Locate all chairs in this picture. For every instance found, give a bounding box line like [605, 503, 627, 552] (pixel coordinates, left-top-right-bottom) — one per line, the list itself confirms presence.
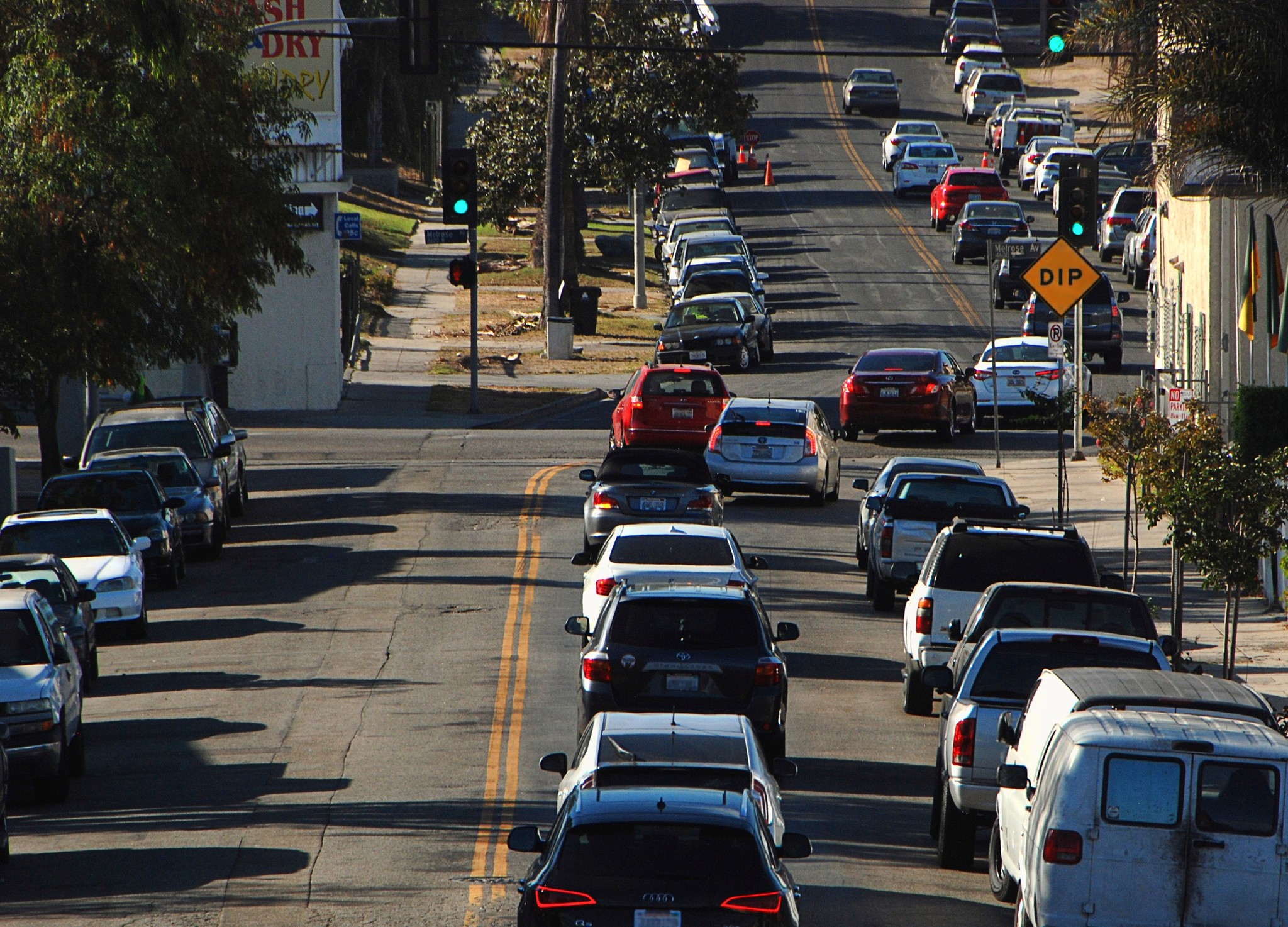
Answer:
[26, 579, 54, 602]
[691, 380, 709, 395]
[156, 461, 182, 486]
[996, 350, 1011, 361]
[936, 153, 960, 157]
[682, 314, 696, 324]
[879, 75, 889, 83]
[912, 149, 921, 156]
[621, 465, 644, 475]
[997, 611, 1032, 629]
[1217, 771, 1273, 827]
[720, 307, 734, 320]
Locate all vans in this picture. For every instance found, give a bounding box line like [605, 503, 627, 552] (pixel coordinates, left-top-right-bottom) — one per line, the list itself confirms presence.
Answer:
[985, 667, 1288, 926]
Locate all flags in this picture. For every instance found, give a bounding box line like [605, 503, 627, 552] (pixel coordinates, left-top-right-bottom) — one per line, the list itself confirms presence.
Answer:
[1238, 204, 1262, 342]
[1265, 214, 1288, 354]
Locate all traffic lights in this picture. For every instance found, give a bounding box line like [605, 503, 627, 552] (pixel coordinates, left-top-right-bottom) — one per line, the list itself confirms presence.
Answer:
[1045, 0, 1081, 64]
[443, 148, 479, 223]
[448, 260, 471, 286]
[1059, 177, 1098, 246]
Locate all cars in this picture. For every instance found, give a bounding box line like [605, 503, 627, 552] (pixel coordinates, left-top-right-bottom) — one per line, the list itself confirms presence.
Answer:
[1018, 137, 1156, 290]
[880, 119, 1035, 264]
[505, 362, 848, 927]
[646, 128, 775, 371]
[840, 337, 1094, 441]
[928, 0, 1028, 146]
[842, 68, 903, 116]
[851, 454, 989, 569]
[0, 397, 253, 797]
[509, 361, 845, 927]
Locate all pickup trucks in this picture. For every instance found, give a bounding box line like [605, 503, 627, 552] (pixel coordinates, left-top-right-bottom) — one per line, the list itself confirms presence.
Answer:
[863, 471, 1031, 613]
[916, 582, 1185, 867]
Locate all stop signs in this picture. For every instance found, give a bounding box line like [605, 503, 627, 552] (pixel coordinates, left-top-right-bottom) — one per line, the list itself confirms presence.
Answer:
[743, 130, 761, 146]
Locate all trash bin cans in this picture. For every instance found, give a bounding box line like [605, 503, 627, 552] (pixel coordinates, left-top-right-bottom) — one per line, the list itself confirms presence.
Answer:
[568, 285, 602, 337]
[546, 317, 574, 360]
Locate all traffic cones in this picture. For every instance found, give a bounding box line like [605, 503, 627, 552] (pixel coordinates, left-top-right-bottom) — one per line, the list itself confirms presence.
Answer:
[981, 150, 989, 167]
[1019, 126, 1026, 143]
[736, 143, 758, 169]
[764, 154, 776, 187]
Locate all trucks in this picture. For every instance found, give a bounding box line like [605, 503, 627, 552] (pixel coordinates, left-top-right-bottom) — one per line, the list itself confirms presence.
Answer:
[999, 95, 1081, 177]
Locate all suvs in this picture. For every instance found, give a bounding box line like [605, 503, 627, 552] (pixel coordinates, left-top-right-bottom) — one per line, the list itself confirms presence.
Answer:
[1022, 270, 1129, 368]
[901, 515, 1126, 717]
